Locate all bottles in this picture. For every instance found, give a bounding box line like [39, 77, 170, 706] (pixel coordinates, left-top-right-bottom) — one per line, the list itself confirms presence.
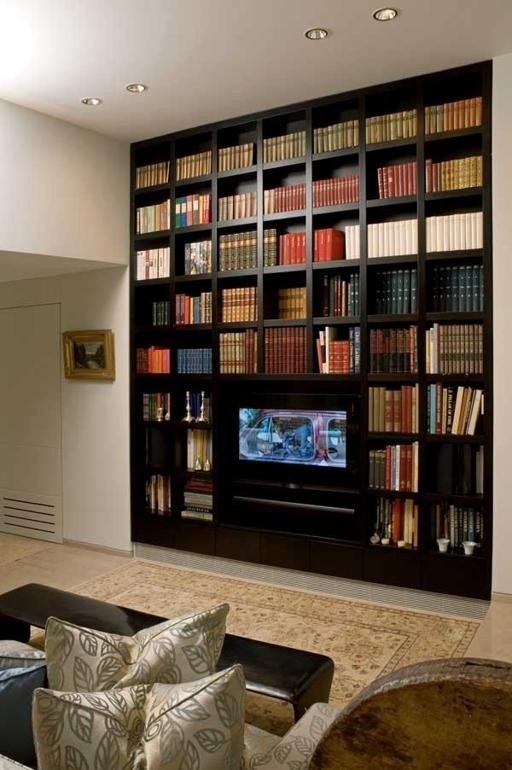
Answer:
[370, 533, 380, 544]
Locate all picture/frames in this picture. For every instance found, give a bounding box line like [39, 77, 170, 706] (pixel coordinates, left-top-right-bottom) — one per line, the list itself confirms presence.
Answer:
[59, 324, 120, 384]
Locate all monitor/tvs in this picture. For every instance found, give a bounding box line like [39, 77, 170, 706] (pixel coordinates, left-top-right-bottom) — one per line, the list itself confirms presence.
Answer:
[232, 394, 361, 494]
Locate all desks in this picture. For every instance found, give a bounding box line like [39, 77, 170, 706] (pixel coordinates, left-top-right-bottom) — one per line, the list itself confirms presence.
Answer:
[0, 578, 333, 725]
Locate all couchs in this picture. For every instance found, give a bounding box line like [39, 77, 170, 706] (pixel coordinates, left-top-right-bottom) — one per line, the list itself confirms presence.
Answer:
[0, 632, 344, 769]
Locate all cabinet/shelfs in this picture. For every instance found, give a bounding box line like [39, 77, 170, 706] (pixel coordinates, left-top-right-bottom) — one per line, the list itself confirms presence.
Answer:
[122, 54, 496, 606]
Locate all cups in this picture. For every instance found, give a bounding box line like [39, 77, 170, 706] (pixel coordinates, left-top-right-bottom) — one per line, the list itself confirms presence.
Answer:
[435, 538, 451, 554]
[398, 540, 406, 548]
[381, 538, 390, 545]
[461, 540, 476, 557]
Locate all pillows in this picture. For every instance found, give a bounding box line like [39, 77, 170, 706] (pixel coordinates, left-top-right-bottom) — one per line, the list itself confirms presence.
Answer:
[40, 603, 230, 694]
[23, 658, 252, 769]
[0, 657, 46, 769]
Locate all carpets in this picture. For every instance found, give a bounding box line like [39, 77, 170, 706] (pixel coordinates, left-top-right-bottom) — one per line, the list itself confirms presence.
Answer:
[60, 558, 482, 706]
